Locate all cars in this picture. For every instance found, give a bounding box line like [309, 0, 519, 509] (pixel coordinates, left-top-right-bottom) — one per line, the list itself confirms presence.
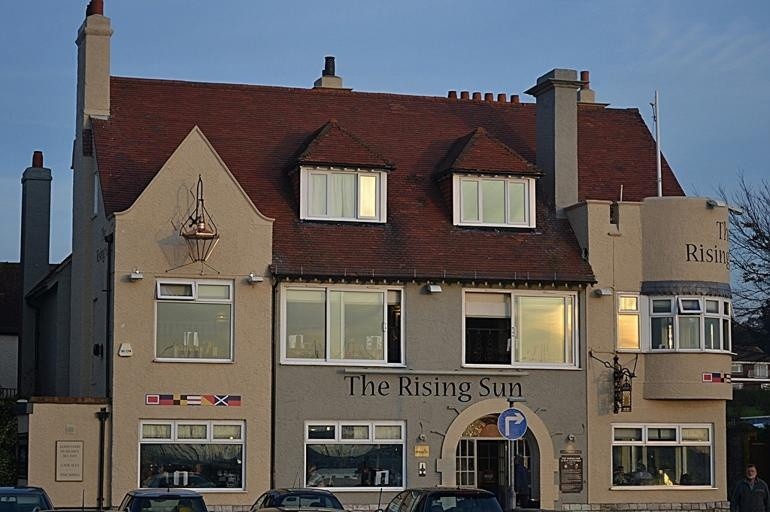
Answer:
[249, 488, 349, 512]
[226, 475, 236, 483]
[373, 485, 504, 511]
[117, 486, 209, 511]
[306, 466, 365, 487]
[143, 472, 214, 490]
[0, 486, 55, 511]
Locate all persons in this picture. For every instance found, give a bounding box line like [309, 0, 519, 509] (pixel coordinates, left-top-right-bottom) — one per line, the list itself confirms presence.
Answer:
[730, 463, 770, 512]
[306, 462, 327, 488]
[613, 453, 711, 486]
[513, 452, 531, 509]
